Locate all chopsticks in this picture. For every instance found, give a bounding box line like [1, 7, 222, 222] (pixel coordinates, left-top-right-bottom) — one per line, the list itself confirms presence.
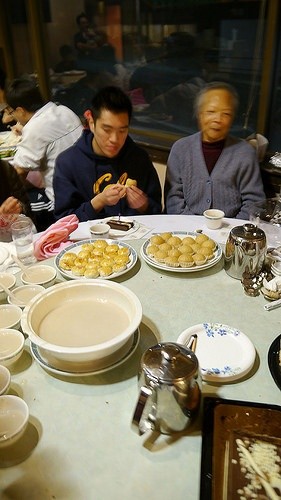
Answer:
[5, 121, 20, 141]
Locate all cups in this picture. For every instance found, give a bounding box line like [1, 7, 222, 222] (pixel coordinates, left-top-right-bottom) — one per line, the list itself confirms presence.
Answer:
[0, 396, 29, 446]
[0, 304, 22, 329]
[241, 270, 265, 296]
[21, 265, 57, 289]
[89, 224, 110, 240]
[1, 272, 45, 307]
[0, 328, 25, 366]
[203, 208, 225, 228]
[0, 365, 12, 395]
[11, 220, 38, 264]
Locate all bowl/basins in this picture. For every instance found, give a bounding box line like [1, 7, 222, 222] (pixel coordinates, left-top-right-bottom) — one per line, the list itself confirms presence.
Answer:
[270, 259, 281, 277]
[20, 279, 143, 362]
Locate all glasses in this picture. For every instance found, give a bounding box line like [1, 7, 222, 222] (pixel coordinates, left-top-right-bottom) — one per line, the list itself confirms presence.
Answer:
[8, 107, 16, 117]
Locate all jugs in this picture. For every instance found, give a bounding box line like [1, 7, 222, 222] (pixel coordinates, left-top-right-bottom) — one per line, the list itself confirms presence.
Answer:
[224, 222, 267, 279]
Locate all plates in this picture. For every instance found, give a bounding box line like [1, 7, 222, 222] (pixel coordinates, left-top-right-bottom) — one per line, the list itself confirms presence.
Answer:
[54, 238, 138, 280]
[31, 329, 141, 377]
[141, 231, 223, 271]
[99, 221, 140, 236]
[0, 131, 21, 160]
[267, 335, 281, 390]
[175, 323, 258, 383]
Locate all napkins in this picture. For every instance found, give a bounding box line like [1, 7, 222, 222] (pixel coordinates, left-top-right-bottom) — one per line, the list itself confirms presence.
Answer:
[32, 214, 80, 260]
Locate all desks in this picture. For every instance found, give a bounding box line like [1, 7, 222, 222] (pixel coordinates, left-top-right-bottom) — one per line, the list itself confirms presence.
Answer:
[0, 215, 281, 500]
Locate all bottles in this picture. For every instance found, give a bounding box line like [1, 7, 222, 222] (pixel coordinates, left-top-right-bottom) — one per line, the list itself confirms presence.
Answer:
[131, 334, 200, 437]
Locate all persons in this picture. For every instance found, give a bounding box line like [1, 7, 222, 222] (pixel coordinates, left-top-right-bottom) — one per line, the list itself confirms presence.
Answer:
[53, 86, 162, 223]
[0, 78, 84, 224]
[163, 82, 265, 221]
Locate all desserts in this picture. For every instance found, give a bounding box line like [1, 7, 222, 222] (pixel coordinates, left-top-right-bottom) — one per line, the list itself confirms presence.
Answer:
[146, 233, 217, 268]
[125, 179, 137, 188]
[60, 239, 131, 278]
